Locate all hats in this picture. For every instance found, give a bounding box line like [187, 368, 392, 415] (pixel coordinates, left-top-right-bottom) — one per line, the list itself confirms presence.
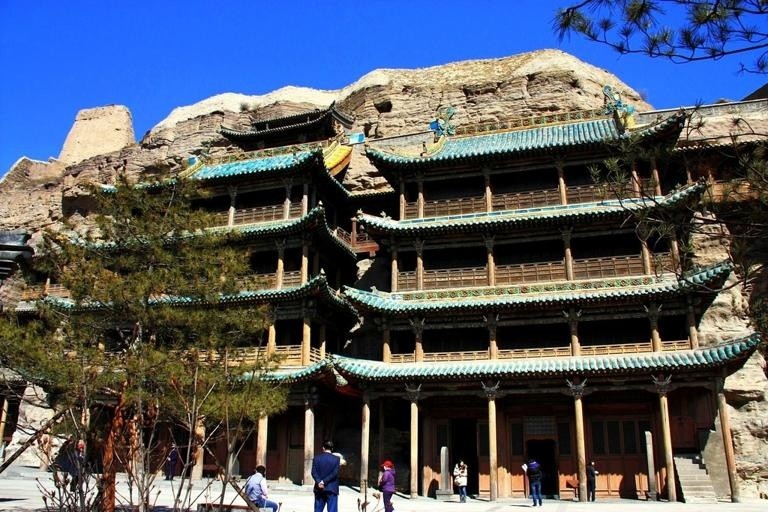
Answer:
[380, 460, 394, 468]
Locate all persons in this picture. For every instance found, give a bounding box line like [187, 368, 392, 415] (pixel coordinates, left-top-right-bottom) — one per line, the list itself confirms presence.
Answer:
[311, 440, 340, 512]
[164, 444, 176, 480]
[378, 460, 395, 512]
[55, 436, 85, 493]
[526, 458, 543, 506]
[245, 465, 278, 512]
[453, 459, 468, 503]
[586, 460, 599, 501]
